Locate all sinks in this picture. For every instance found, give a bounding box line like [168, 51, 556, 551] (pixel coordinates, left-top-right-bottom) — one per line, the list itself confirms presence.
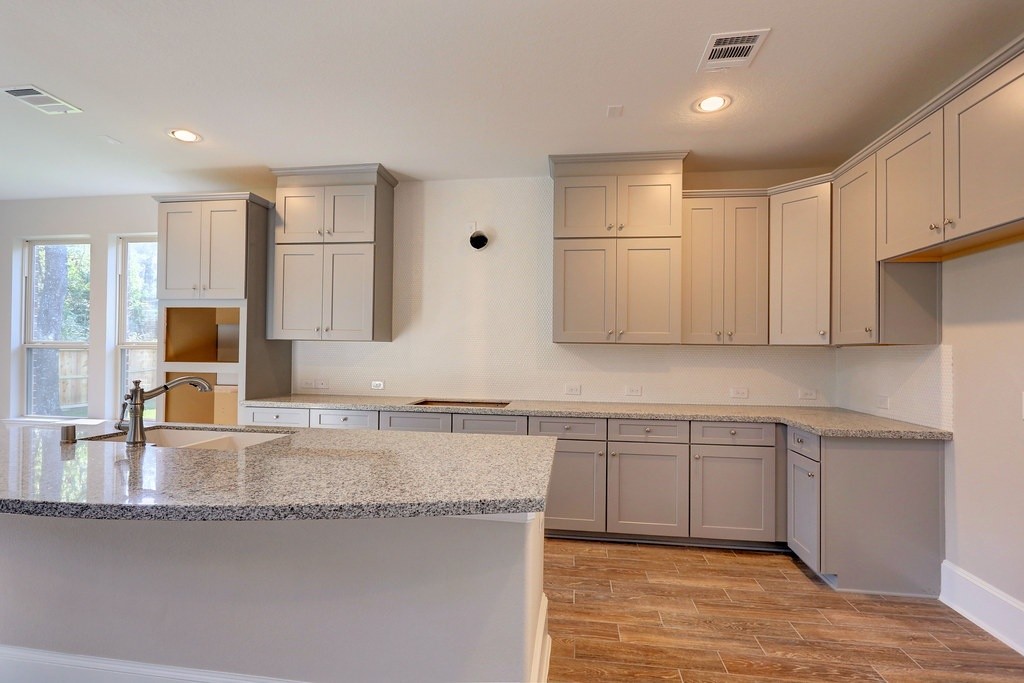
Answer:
[181, 430, 299, 450]
[78, 425, 226, 448]
[406, 397, 510, 409]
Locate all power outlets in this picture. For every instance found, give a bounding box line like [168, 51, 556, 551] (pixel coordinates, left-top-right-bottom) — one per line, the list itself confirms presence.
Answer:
[730, 386, 748, 399]
[877, 396, 890, 410]
[371, 380, 385, 389]
[564, 383, 581, 395]
[625, 384, 643, 396]
[798, 387, 818, 399]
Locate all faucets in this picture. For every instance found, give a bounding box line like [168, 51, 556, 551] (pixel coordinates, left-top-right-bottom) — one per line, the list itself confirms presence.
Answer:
[113, 374, 211, 449]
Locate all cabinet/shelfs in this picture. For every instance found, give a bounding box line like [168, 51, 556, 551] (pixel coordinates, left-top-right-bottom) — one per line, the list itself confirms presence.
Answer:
[242, 408, 380, 433]
[547, 147, 688, 238]
[787, 424, 819, 584]
[765, 185, 832, 350]
[380, 412, 528, 435]
[153, 190, 272, 424]
[267, 162, 398, 344]
[528, 417, 691, 540]
[549, 242, 682, 345]
[876, 54, 1024, 264]
[689, 422, 776, 543]
[832, 151, 941, 346]
[681, 188, 771, 345]
